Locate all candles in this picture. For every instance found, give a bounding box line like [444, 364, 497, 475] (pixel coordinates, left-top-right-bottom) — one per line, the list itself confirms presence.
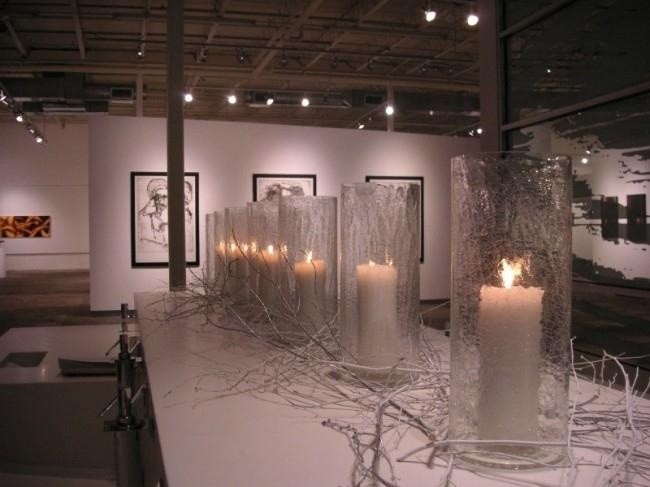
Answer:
[212, 242, 400, 381]
[470, 257, 544, 457]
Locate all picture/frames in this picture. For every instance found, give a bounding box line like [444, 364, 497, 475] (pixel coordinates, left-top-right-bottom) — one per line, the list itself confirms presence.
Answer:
[252, 172, 317, 203]
[129, 171, 201, 267]
[364, 174, 424, 264]
